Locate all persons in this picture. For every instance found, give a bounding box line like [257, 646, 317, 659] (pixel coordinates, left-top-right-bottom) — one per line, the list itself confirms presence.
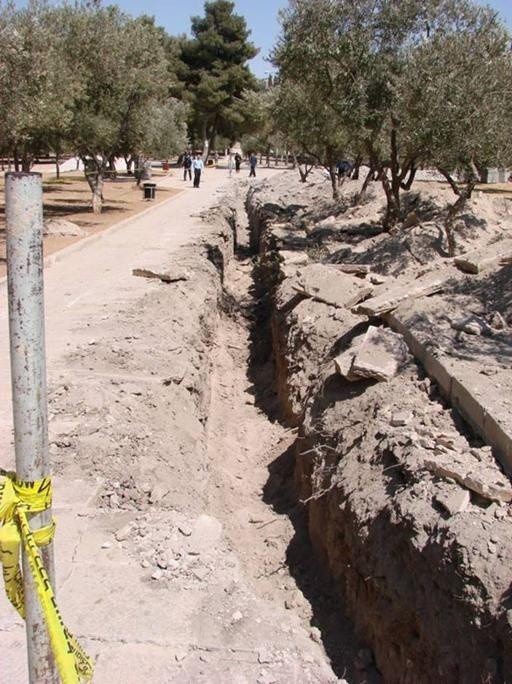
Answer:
[234, 152, 242, 172]
[247, 151, 256, 177]
[192, 153, 205, 188]
[181, 153, 193, 181]
[214, 150, 220, 165]
[227, 155, 234, 179]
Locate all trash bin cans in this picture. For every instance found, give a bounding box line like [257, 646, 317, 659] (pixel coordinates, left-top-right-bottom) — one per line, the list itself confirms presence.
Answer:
[143, 183, 155, 198]
[161, 159, 168, 169]
